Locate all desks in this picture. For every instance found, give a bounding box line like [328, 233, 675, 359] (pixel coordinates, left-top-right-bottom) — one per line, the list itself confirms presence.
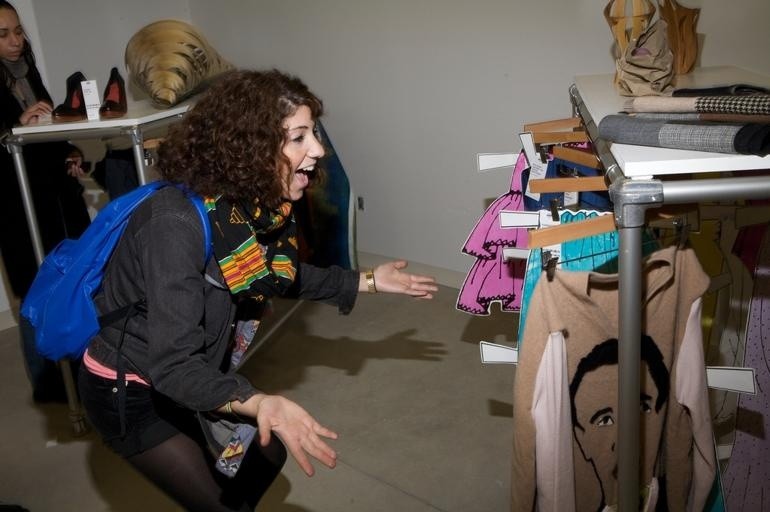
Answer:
[7, 95, 191, 448]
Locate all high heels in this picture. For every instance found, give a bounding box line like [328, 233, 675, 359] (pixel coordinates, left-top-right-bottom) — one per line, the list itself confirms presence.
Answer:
[99, 67, 127, 118]
[51, 71, 88, 125]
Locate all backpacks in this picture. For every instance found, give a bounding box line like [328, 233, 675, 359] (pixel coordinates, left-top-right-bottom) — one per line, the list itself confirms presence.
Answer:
[19, 180, 213, 362]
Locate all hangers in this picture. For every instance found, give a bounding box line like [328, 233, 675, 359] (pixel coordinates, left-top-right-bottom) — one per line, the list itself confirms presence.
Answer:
[522, 84, 701, 281]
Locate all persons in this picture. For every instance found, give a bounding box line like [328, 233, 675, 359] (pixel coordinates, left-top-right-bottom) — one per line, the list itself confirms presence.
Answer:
[0, 0, 93, 404]
[75, 67, 440, 511]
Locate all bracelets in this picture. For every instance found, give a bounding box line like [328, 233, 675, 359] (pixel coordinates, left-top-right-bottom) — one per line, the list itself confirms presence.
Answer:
[365, 269, 377, 295]
[225, 400, 233, 415]
